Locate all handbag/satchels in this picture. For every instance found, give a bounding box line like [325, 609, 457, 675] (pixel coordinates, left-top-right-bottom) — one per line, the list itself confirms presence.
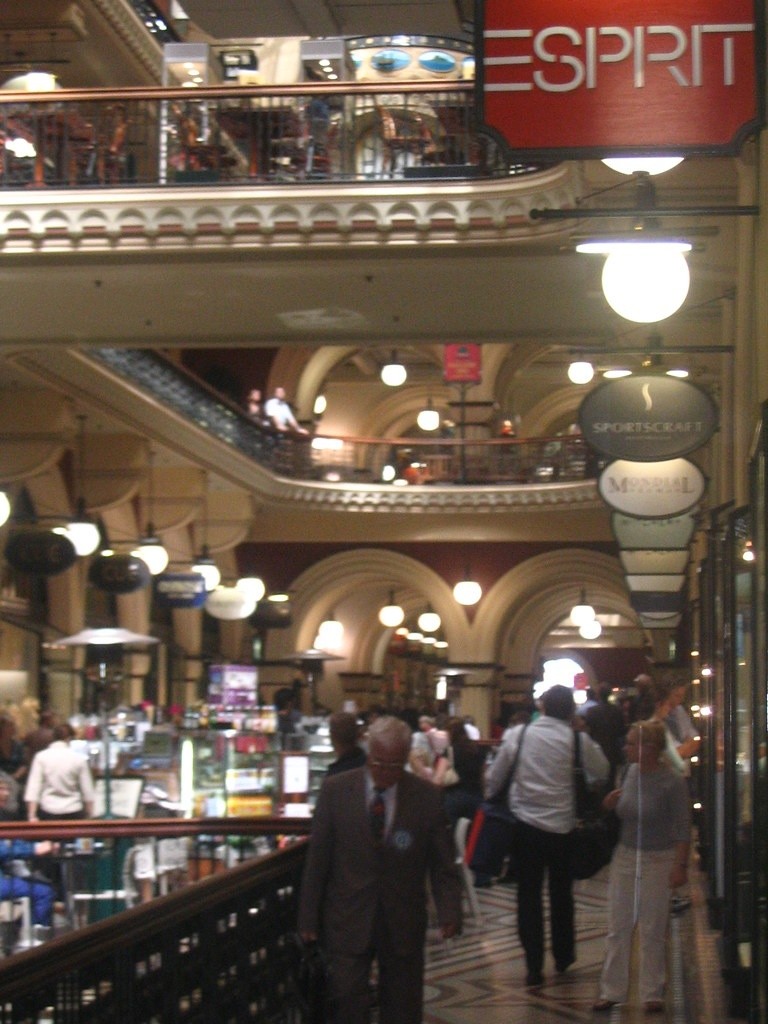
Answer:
[566, 816, 613, 879]
[439, 747, 460, 787]
[464, 803, 516, 870]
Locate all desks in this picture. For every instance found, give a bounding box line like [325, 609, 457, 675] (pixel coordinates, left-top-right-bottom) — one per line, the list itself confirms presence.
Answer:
[0, 108, 95, 187]
[207, 104, 300, 176]
[431, 99, 475, 164]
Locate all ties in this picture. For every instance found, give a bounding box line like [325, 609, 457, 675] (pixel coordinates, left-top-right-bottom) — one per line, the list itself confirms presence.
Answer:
[368, 786, 387, 842]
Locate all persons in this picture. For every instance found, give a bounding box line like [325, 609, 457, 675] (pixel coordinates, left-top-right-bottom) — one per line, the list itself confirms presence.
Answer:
[483, 686, 608, 986]
[0, 671, 704, 934]
[240, 387, 278, 428]
[261, 387, 308, 470]
[595, 719, 693, 1010]
[295, 716, 466, 1024]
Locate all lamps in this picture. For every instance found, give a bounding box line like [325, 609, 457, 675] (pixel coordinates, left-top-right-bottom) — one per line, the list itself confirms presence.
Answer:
[569, 588, 602, 641]
[576, 170, 692, 322]
[568, 353, 594, 384]
[380, 351, 407, 387]
[45, 415, 266, 620]
[417, 398, 441, 431]
[378, 558, 485, 648]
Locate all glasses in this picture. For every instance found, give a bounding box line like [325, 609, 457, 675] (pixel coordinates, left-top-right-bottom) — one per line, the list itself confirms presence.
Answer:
[369, 762, 403, 773]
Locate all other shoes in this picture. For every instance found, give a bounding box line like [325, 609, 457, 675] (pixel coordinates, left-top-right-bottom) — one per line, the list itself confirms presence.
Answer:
[669, 895, 690, 912]
[647, 1001, 664, 1011]
[594, 998, 617, 1010]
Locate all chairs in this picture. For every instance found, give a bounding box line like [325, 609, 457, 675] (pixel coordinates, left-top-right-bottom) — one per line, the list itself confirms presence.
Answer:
[68, 92, 427, 184]
